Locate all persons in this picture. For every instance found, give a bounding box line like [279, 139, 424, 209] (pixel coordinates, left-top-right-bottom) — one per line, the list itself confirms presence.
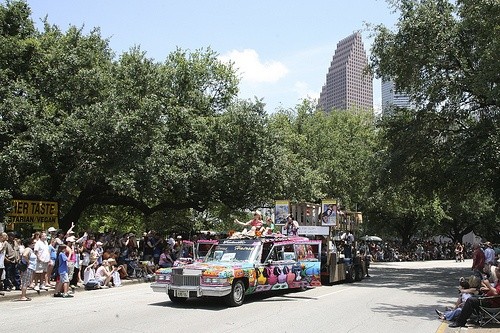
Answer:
[232, 210, 264, 233]
[0, 219, 500, 329]
[264, 215, 299, 237]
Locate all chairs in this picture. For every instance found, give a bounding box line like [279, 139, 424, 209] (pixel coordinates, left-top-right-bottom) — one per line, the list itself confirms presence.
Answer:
[478, 295, 500, 326]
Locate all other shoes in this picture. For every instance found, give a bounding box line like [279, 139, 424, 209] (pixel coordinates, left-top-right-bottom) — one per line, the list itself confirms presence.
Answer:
[53, 291, 63, 297]
[449, 320, 466, 327]
[39, 284, 48, 290]
[44, 284, 52, 287]
[15, 287, 21, 291]
[19, 296, 32, 301]
[63, 293, 74, 298]
[434, 308, 446, 320]
[49, 282, 57, 286]
[366, 275, 371, 277]
[34, 286, 40, 292]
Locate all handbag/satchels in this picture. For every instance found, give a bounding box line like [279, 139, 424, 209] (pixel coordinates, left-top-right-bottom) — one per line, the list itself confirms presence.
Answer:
[16, 260, 28, 272]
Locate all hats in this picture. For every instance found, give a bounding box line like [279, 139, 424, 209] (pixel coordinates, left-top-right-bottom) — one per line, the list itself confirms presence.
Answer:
[483, 241, 492, 248]
[96, 241, 104, 246]
[70, 235, 78, 240]
[47, 226, 57, 232]
[67, 230, 75, 234]
[66, 237, 76, 243]
[108, 257, 116, 261]
[251, 210, 262, 220]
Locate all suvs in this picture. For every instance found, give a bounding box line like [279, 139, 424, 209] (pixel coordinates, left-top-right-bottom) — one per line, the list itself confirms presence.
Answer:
[150, 236, 322, 305]
